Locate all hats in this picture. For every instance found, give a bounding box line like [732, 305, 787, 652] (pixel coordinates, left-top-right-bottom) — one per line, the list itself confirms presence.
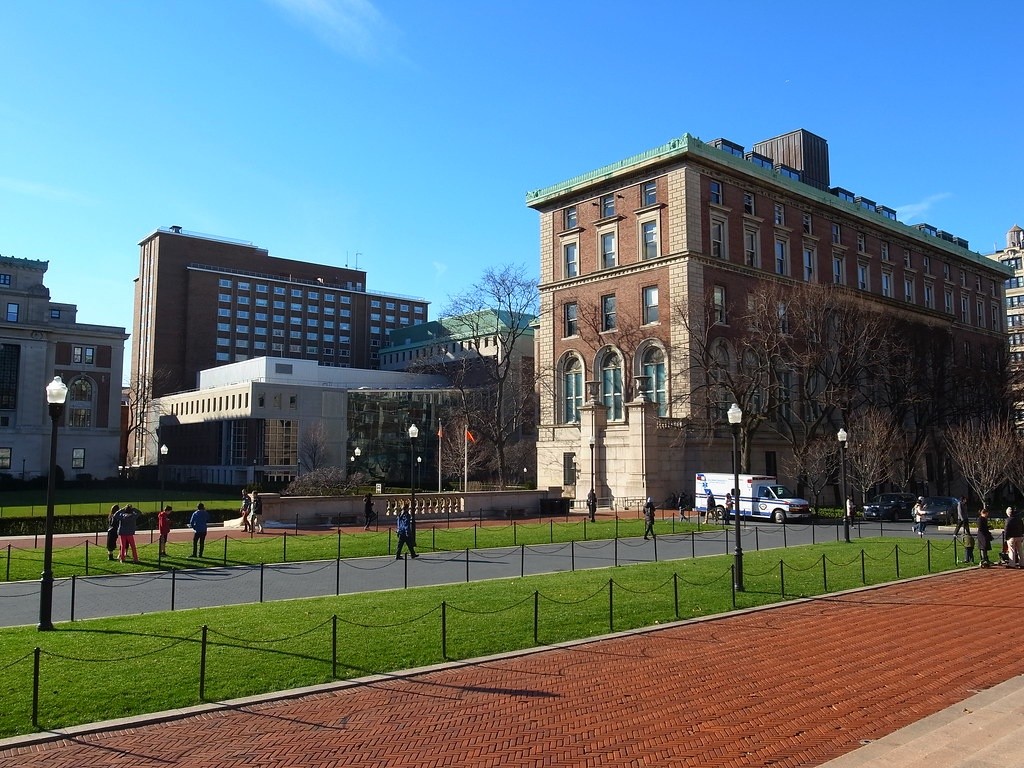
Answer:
[647, 497, 653, 503]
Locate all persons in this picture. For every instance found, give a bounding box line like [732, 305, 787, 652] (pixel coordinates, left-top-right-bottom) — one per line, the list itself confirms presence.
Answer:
[702, 491, 719, 525]
[977, 509, 994, 564]
[725, 493, 735, 524]
[642, 496, 656, 540]
[364, 492, 376, 530]
[587, 489, 598, 518]
[189, 503, 208, 557]
[846, 494, 855, 529]
[251, 490, 264, 533]
[911, 495, 930, 534]
[1004, 506, 1024, 568]
[678, 492, 688, 522]
[961, 530, 975, 563]
[113, 504, 142, 563]
[158, 505, 172, 557]
[240, 489, 251, 533]
[952, 496, 972, 538]
[395, 504, 419, 560]
[106, 505, 120, 561]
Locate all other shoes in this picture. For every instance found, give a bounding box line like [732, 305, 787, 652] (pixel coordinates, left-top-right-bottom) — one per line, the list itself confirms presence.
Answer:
[412, 554, 419, 558]
[918, 531, 924, 535]
[953, 535, 957, 537]
[190, 555, 197, 557]
[397, 557, 403, 560]
[1016, 564, 1024, 569]
[160, 553, 168, 557]
[1006, 565, 1015, 569]
[912, 527, 916, 532]
[110, 558, 118, 561]
[852, 527, 856, 529]
[133, 561, 140, 564]
[643, 537, 649, 540]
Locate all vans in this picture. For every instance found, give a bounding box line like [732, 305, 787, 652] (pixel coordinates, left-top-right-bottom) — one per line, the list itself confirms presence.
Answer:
[863, 492, 918, 522]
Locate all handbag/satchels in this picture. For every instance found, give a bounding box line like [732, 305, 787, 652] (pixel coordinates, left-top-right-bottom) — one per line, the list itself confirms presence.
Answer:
[911, 505, 918, 516]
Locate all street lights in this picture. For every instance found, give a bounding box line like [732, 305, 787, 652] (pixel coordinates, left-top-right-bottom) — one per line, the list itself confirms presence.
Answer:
[416, 455, 422, 492]
[408, 424, 419, 546]
[37, 376, 68, 631]
[351, 446, 361, 495]
[524, 468, 527, 485]
[727, 403, 746, 592]
[589, 437, 595, 490]
[572, 456, 578, 498]
[297, 459, 301, 476]
[254, 460, 256, 485]
[837, 428, 852, 543]
[160, 443, 168, 512]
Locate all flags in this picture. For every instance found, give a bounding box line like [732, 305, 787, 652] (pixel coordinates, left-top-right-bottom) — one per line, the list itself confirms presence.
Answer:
[467, 431, 475, 443]
[438, 425, 442, 437]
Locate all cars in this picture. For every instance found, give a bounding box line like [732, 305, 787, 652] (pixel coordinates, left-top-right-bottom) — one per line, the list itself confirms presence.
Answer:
[921, 496, 959, 525]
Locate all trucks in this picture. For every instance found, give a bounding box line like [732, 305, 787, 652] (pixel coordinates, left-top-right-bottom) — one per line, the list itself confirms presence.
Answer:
[692, 472, 811, 524]
